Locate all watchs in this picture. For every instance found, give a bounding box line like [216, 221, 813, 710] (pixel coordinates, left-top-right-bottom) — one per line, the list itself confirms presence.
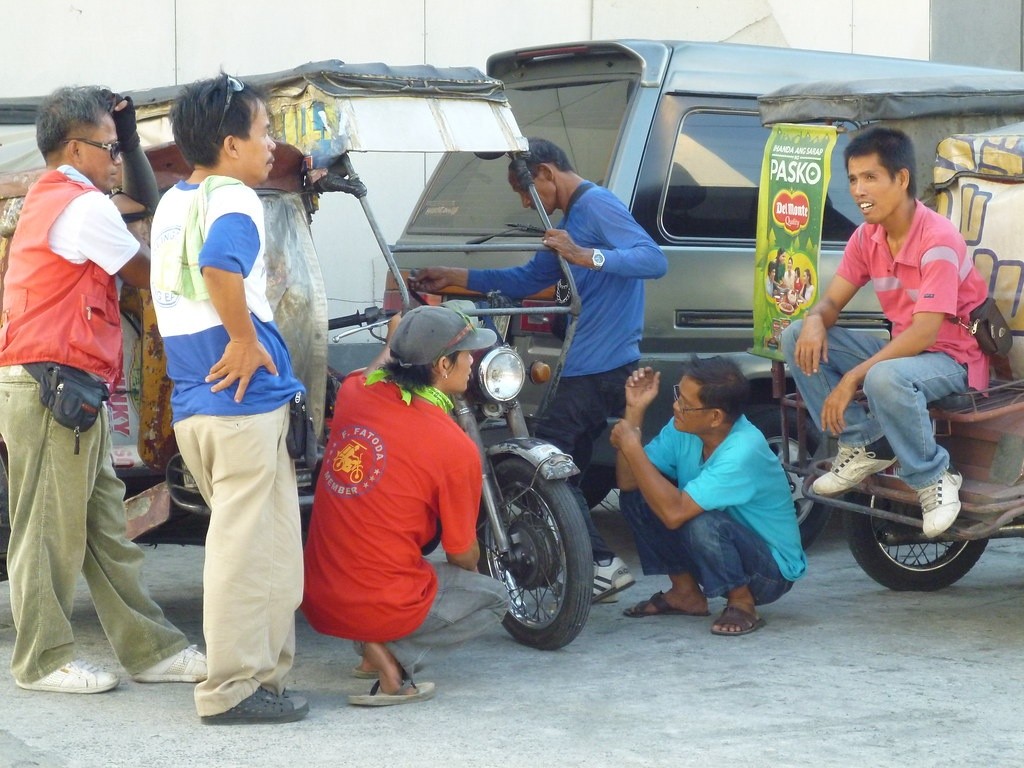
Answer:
[592, 248, 605, 271]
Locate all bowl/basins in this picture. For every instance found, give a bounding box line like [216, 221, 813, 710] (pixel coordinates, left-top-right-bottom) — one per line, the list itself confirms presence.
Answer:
[777, 285, 804, 314]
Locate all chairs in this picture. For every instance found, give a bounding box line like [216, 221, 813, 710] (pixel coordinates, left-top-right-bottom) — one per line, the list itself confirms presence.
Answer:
[663, 160, 707, 240]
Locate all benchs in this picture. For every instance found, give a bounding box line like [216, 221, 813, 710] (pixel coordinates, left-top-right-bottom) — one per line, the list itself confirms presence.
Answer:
[813, 375, 1024, 539]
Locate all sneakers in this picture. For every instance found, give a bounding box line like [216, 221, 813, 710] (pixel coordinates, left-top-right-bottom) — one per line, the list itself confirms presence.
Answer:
[597, 592, 619, 604]
[812, 435, 897, 498]
[200, 685, 309, 725]
[128, 646, 208, 683]
[914, 462, 963, 538]
[16, 658, 121, 694]
[591, 556, 636, 605]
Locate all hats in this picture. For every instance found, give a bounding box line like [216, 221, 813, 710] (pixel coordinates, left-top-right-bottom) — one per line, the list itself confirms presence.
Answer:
[389, 305, 497, 370]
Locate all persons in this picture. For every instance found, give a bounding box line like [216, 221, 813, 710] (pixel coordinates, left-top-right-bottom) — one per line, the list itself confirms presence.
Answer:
[409, 137, 669, 608]
[150, 73, 311, 726]
[1, 87, 211, 695]
[780, 129, 1012, 537]
[610, 355, 809, 636]
[300, 307, 510, 706]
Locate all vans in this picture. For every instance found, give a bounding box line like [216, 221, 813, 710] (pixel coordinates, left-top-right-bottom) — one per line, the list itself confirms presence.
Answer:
[384, 37, 1024, 513]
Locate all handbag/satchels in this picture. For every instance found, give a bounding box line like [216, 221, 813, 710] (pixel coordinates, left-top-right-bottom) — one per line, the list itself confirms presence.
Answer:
[285, 390, 317, 472]
[969, 296, 1013, 357]
[21, 362, 110, 432]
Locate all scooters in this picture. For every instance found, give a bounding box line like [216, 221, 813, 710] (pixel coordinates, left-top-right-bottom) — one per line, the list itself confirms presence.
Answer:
[0, 58, 595, 649]
[771, 310, 1023, 593]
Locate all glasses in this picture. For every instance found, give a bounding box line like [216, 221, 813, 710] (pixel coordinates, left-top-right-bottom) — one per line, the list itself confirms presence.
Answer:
[213, 75, 245, 143]
[433, 310, 478, 367]
[673, 385, 730, 414]
[62, 138, 122, 160]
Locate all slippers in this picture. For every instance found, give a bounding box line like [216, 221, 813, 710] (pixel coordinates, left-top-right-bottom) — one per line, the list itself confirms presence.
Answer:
[711, 606, 767, 636]
[347, 663, 436, 706]
[622, 590, 712, 618]
